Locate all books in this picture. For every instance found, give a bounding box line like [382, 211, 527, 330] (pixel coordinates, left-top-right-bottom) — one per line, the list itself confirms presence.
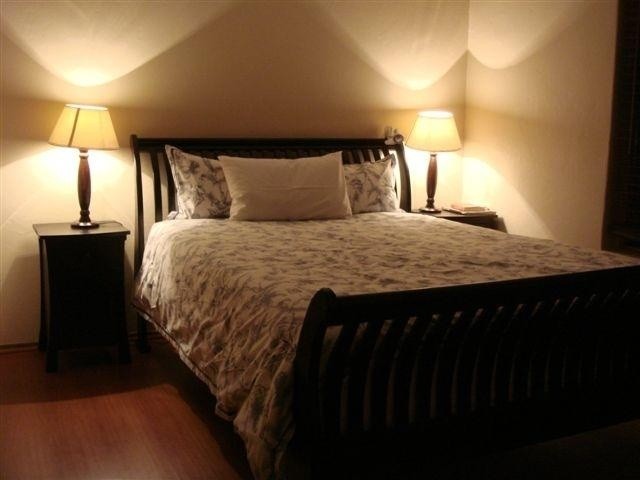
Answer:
[450, 202, 485, 212]
[441, 206, 497, 217]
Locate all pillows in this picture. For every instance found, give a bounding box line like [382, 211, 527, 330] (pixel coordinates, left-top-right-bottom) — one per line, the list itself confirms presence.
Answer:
[165, 144, 229, 221]
[219, 151, 352, 221]
[343, 155, 402, 213]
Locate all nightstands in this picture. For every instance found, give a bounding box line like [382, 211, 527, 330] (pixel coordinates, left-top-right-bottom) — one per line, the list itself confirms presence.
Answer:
[33, 221, 131, 373]
[413, 209, 498, 229]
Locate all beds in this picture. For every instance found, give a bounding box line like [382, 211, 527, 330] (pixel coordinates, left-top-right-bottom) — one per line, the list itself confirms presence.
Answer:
[129, 134, 640, 480]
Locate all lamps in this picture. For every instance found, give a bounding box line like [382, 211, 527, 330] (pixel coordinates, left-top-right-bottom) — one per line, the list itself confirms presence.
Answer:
[405, 112, 462, 213]
[49, 104, 120, 228]
